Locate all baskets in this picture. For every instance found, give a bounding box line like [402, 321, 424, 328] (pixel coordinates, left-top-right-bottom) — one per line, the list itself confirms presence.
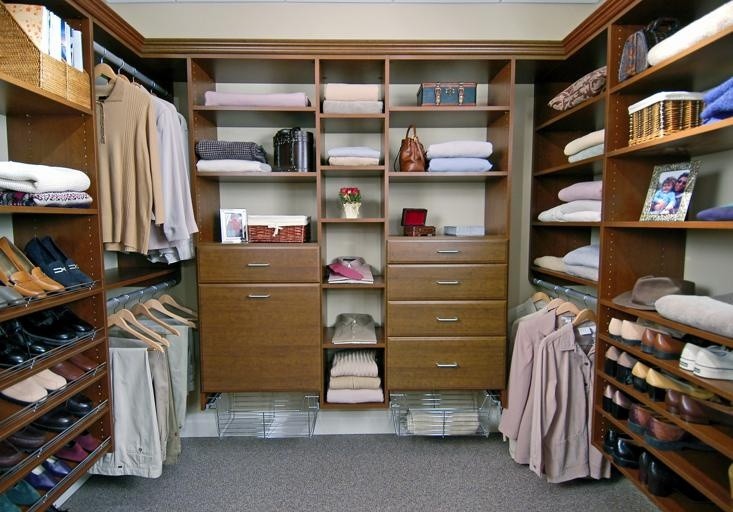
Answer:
[627, 91, 707, 147]
[388, 390, 502, 439]
[248, 215, 311, 244]
[214, 393, 319, 439]
[0, 2, 90, 110]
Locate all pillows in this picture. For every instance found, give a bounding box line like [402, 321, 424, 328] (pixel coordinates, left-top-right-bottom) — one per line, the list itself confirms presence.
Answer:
[546, 66, 607, 111]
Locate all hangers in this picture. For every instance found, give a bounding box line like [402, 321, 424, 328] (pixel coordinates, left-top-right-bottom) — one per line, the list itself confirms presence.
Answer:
[92, 44, 174, 111]
[105, 282, 196, 352]
[531, 280, 597, 329]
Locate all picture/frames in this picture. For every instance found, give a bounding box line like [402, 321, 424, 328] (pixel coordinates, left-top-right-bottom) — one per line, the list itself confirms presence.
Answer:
[638, 159, 704, 222]
[220, 208, 249, 245]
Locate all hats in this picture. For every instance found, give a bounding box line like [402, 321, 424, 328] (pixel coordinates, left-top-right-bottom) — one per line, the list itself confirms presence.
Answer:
[611, 275, 697, 313]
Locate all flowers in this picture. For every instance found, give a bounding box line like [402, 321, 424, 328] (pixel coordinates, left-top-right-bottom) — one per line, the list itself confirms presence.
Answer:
[336, 187, 362, 211]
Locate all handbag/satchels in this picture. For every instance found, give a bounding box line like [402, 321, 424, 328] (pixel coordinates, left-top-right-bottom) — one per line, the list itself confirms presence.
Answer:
[273, 127, 313, 173]
[399, 137, 426, 171]
[618, 17, 680, 82]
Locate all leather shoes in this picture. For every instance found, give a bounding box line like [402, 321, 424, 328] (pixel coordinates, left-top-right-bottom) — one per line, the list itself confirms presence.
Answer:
[0, 235, 99, 512]
[602, 318, 732, 498]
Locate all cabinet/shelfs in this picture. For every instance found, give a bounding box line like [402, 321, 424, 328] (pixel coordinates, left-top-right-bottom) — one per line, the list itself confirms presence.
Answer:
[382, 54, 511, 404]
[188, 53, 322, 403]
[0, 0, 114, 512]
[313, 56, 389, 409]
[529, 0, 733, 511]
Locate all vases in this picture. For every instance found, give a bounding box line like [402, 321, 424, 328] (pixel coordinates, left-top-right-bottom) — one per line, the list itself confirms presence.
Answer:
[344, 204, 360, 219]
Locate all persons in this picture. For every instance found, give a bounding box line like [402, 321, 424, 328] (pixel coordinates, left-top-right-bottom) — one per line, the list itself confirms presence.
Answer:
[227, 213, 241, 237]
[651, 177, 676, 214]
[672, 173, 688, 212]
